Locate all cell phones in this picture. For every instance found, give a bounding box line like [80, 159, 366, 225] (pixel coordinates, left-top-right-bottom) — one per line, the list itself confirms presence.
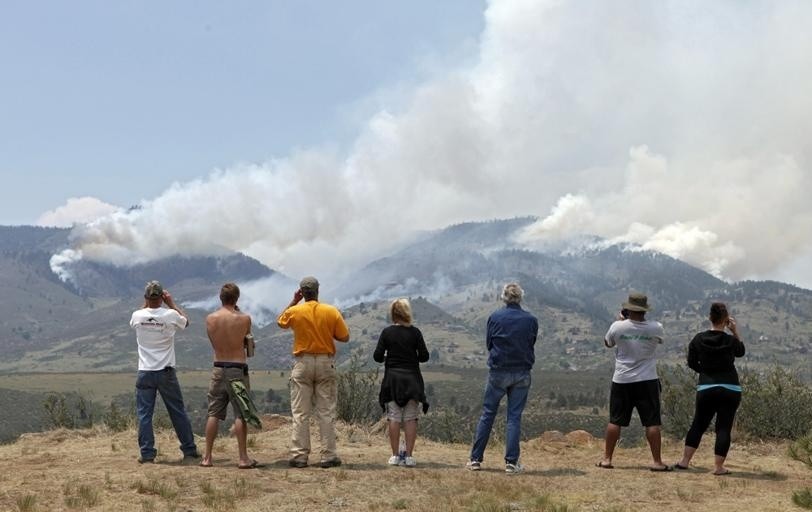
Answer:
[727, 319, 729, 326]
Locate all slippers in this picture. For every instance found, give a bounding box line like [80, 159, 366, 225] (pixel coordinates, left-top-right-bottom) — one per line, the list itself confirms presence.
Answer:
[238, 458, 264, 469]
[595, 461, 614, 468]
[198, 459, 213, 467]
[713, 468, 733, 475]
[649, 464, 674, 472]
[674, 462, 689, 469]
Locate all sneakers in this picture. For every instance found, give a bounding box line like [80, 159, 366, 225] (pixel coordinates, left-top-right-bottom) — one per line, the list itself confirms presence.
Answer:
[137, 455, 155, 465]
[320, 456, 342, 469]
[182, 453, 202, 466]
[404, 456, 417, 466]
[504, 460, 521, 476]
[388, 455, 401, 466]
[287, 457, 308, 469]
[464, 458, 481, 472]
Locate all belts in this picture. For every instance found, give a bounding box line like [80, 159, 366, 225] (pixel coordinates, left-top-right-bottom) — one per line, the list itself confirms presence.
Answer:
[212, 363, 246, 369]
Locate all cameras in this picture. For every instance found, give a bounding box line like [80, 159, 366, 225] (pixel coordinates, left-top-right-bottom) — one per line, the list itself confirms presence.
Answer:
[621, 309, 629, 319]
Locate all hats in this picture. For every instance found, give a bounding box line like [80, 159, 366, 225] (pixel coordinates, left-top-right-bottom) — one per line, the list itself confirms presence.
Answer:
[299, 275, 319, 296]
[143, 280, 164, 301]
[621, 293, 653, 312]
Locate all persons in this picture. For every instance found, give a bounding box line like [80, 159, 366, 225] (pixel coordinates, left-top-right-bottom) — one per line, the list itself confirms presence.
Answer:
[595, 293, 673, 472]
[466, 282, 538, 475]
[373, 298, 430, 467]
[129, 281, 202, 464]
[198, 282, 264, 469]
[675, 303, 746, 475]
[276, 276, 349, 468]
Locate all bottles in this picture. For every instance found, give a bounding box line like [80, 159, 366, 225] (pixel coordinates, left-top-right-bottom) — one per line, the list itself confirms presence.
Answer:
[245, 333, 254, 357]
